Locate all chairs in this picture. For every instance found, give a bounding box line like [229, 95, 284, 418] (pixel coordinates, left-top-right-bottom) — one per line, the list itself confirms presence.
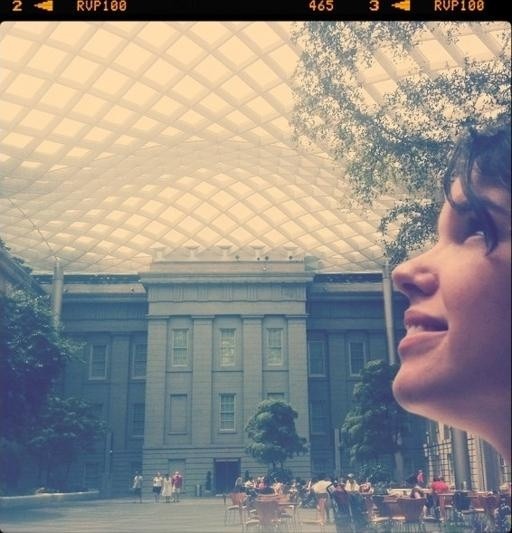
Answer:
[222, 482, 501, 533]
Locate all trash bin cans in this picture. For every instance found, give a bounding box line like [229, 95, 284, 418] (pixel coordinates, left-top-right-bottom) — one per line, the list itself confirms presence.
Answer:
[195, 484, 202, 497]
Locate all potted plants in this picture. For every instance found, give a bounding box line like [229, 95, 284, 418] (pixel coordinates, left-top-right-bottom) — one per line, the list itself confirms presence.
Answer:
[204, 471, 212, 496]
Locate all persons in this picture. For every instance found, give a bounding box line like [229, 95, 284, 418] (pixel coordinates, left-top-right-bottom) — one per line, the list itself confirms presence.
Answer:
[152, 472, 163, 503]
[133, 471, 143, 504]
[161, 473, 172, 503]
[171, 472, 183, 502]
[393, 123, 512, 468]
[231, 467, 512, 532]
